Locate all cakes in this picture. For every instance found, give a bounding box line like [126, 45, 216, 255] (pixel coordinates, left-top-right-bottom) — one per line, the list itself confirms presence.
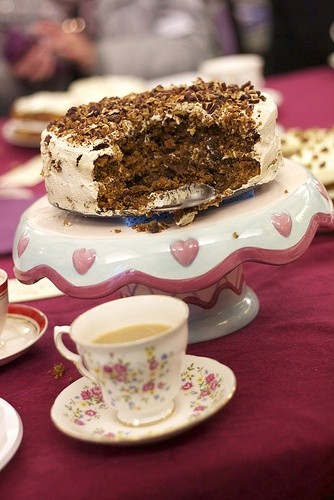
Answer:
[39, 78, 280, 220]
[274, 124, 334, 198]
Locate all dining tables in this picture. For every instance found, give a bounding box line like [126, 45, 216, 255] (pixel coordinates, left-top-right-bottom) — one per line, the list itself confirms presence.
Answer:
[0, 68, 334, 500]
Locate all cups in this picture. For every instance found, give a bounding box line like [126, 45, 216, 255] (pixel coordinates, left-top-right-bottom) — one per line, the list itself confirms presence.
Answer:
[53, 294, 190, 426]
[0, 268, 8, 333]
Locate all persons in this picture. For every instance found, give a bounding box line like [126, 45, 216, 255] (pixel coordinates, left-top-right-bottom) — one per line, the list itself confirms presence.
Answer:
[0, 0, 99, 117]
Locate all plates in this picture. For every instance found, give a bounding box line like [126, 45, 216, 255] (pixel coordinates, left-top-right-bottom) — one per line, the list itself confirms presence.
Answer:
[49, 355, 237, 443]
[0, 398, 23, 471]
[0, 303, 49, 367]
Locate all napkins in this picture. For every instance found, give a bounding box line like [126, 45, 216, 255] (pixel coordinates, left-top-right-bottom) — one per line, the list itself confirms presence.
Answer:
[6, 277, 66, 304]
